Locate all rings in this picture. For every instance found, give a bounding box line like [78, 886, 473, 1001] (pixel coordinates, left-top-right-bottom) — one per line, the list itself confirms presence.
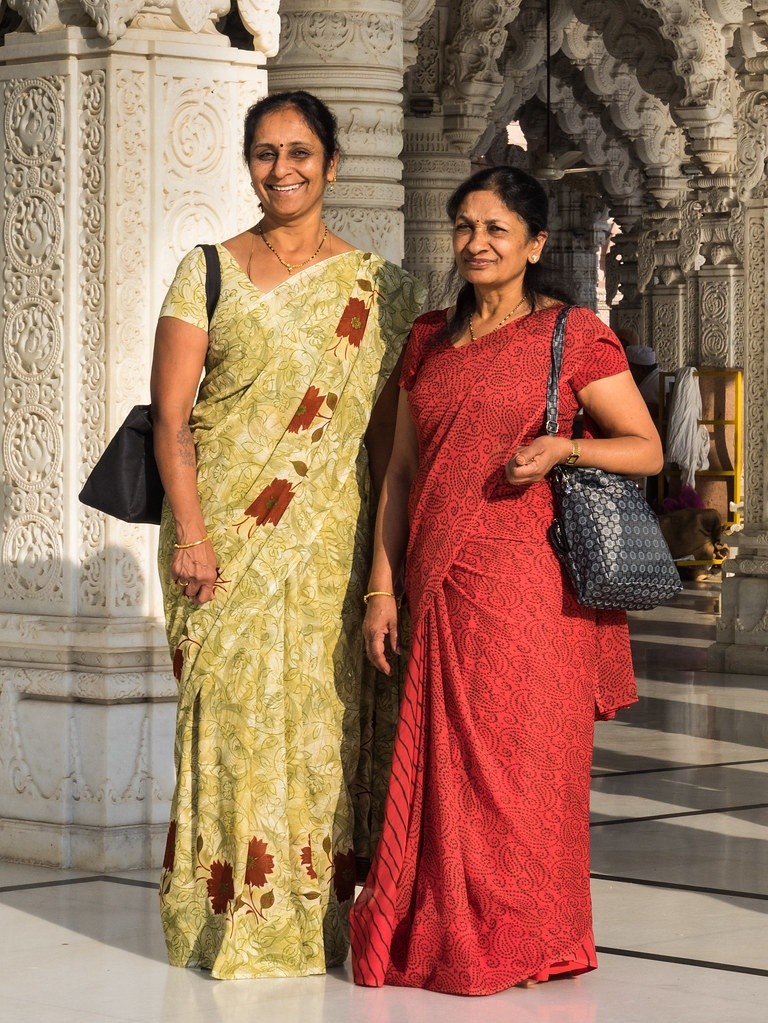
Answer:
[178, 580, 188, 586]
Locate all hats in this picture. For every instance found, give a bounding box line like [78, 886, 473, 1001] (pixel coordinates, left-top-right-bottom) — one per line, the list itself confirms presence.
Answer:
[626, 344, 656, 365]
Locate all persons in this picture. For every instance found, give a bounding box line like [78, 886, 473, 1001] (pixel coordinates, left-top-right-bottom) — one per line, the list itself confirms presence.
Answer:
[626, 344, 675, 407]
[361, 166, 664, 989]
[151, 91, 401, 980]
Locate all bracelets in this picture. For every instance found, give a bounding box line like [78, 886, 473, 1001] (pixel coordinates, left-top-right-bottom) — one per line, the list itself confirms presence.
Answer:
[174, 536, 210, 548]
[364, 592, 395, 601]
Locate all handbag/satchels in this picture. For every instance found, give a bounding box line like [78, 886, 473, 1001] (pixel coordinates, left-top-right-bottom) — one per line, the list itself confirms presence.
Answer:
[540, 302, 682, 611]
[78, 244, 221, 523]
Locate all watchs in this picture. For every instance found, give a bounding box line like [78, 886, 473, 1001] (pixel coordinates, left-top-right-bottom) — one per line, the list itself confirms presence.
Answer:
[565, 439, 580, 466]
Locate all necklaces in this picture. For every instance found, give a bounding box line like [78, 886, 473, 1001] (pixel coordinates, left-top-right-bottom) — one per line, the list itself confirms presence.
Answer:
[258, 221, 328, 275]
[469, 296, 527, 341]
